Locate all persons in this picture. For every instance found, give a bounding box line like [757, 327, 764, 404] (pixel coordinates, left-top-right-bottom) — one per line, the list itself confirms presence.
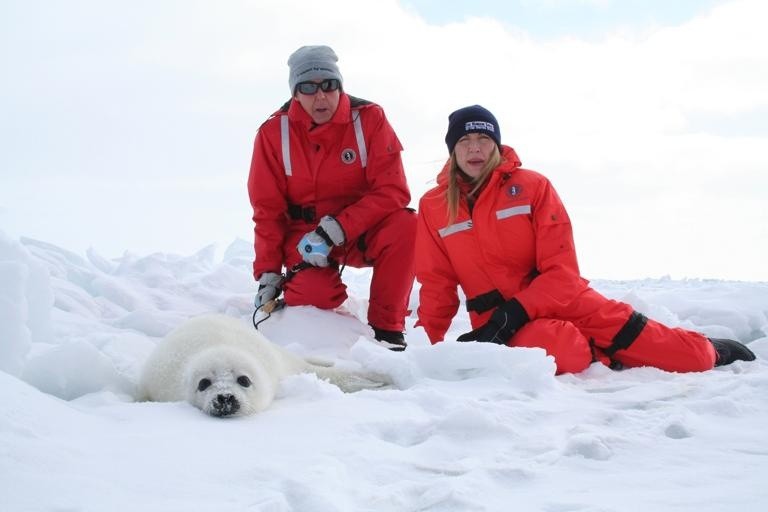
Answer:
[247, 43, 420, 352]
[416, 105, 757, 374]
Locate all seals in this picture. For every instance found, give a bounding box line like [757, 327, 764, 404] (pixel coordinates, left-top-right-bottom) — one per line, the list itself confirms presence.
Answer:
[135, 312, 385, 419]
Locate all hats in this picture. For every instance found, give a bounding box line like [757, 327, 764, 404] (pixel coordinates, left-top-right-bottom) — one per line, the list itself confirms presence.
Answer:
[446, 106, 501, 156]
[287, 45, 343, 100]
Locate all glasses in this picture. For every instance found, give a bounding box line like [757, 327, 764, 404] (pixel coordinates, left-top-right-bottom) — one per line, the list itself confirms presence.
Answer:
[296, 79, 339, 95]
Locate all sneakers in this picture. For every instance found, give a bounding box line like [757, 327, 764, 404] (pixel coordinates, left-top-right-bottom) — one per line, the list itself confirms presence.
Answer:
[709, 337, 756, 366]
[368, 323, 407, 350]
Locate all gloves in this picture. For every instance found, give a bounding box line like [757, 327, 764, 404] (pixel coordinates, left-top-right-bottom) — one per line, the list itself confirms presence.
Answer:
[254, 273, 285, 312]
[296, 215, 346, 268]
[457, 299, 530, 345]
